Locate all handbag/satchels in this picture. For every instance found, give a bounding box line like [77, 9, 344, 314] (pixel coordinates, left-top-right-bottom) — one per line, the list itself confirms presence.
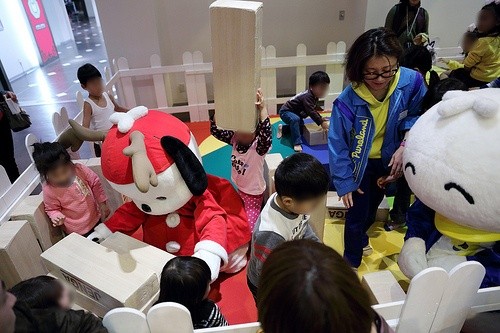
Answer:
[0, 92, 31, 132]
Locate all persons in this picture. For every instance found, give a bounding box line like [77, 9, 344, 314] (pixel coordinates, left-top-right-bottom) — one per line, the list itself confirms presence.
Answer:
[277, 71, 328, 153]
[400, 45, 440, 87]
[77, 63, 131, 157]
[0, 278, 108, 333]
[247, 153, 330, 310]
[156, 255, 230, 330]
[327, 28, 430, 270]
[0, 89, 20, 184]
[29, 142, 111, 245]
[383, 0, 430, 52]
[208, 88, 272, 237]
[10, 275, 71, 311]
[254, 239, 397, 333]
[437, 2, 500, 91]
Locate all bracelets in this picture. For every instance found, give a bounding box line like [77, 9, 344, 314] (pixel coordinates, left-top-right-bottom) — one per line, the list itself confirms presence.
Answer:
[400, 142, 406, 147]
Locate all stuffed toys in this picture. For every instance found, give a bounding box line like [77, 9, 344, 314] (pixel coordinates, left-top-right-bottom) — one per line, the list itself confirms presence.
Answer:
[59, 106, 251, 300]
[397, 88, 500, 289]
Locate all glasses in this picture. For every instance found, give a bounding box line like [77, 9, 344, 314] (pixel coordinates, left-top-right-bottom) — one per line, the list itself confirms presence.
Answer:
[363, 61, 399, 80]
